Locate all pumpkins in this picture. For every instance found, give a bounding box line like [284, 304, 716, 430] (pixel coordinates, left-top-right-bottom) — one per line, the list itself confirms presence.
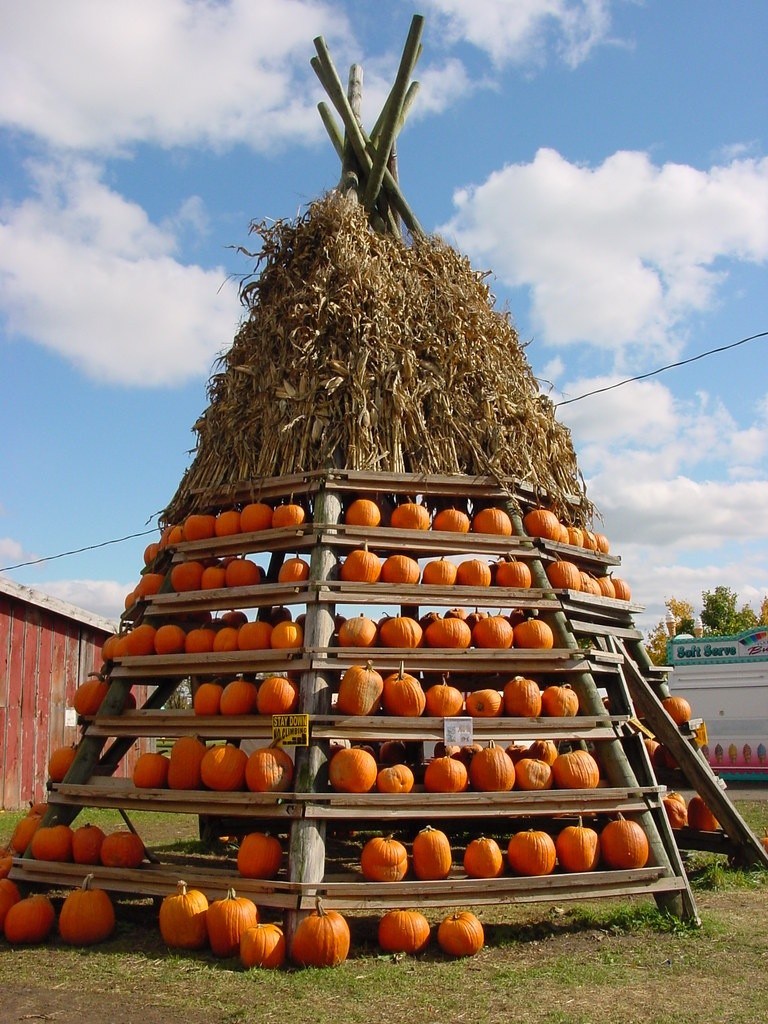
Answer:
[757, 826, 768, 853]
[0, 496, 718, 971]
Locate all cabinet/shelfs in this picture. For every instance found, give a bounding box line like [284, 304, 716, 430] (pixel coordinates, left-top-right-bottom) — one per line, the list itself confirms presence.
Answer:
[8, 469, 768, 958]
